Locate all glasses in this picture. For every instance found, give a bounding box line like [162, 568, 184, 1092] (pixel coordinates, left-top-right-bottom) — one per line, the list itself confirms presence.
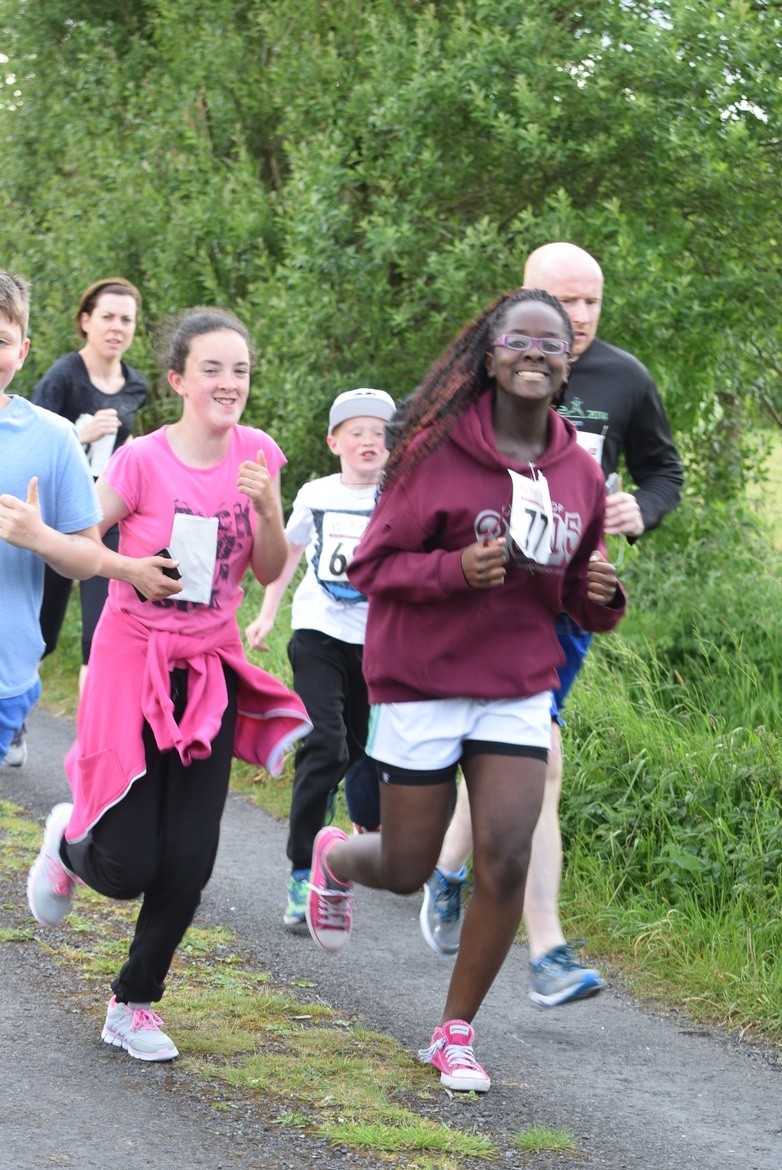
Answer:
[493, 330, 572, 361]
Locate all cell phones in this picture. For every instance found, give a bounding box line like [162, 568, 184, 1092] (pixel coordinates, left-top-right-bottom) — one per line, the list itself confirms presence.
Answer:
[132, 547, 183, 602]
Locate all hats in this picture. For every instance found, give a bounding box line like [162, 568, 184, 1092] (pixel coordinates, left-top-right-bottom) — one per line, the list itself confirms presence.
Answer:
[327, 386, 397, 438]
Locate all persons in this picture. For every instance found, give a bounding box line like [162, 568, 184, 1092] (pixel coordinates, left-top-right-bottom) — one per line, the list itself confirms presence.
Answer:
[245, 389, 398, 933]
[5, 278, 151, 767]
[27, 308, 287, 1061]
[421, 241, 686, 1007]
[306, 288, 627, 1091]
[0, 273, 104, 767]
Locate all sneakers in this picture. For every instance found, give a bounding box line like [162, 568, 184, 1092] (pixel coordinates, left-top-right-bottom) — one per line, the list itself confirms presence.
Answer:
[283, 869, 308, 933]
[5, 728, 30, 770]
[429, 1018, 493, 1092]
[100, 995, 180, 1063]
[529, 945, 606, 1008]
[310, 824, 354, 954]
[24, 800, 79, 928]
[419, 861, 470, 955]
